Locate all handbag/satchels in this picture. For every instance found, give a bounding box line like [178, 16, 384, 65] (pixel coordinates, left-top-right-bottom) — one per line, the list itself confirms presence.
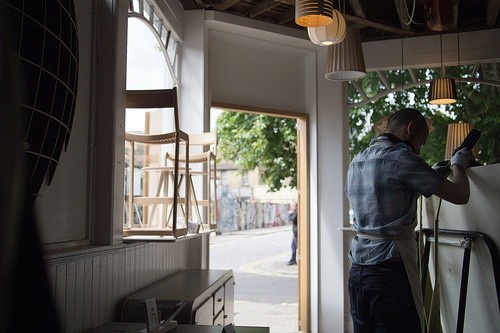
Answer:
[288, 213, 297, 222]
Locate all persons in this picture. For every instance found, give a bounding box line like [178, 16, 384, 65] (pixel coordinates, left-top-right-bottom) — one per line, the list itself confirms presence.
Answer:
[344, 108, 473, 333]
[288, 202, 297, 265]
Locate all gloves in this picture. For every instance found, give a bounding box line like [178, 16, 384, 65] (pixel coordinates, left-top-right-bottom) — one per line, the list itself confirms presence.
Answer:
[451, 147, 475, 171]
[431, 159, 450, 172]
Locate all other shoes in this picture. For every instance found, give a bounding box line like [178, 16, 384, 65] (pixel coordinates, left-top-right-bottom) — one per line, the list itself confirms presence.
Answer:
[288, 259, 296, 265]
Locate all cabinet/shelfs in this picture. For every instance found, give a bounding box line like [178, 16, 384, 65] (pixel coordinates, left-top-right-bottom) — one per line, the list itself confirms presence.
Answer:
[120, 269, 235, 325]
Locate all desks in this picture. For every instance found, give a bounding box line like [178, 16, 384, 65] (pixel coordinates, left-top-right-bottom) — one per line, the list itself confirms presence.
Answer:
[141, 167, 192, 229]
[86, 321, 269, 333]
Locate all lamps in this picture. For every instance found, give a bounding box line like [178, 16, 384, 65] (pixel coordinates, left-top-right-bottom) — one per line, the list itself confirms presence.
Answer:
[325, 0, 367, 81]
[295, 0, 334, 27]
[428, 31, 458, 104]
[307, 8, 348, 46]
[444, 25, 479, 161]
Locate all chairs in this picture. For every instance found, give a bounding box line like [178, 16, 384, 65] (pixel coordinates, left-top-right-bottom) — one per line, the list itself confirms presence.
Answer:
[149, 132, 218, 229]
[124, 88, 190, 238]
